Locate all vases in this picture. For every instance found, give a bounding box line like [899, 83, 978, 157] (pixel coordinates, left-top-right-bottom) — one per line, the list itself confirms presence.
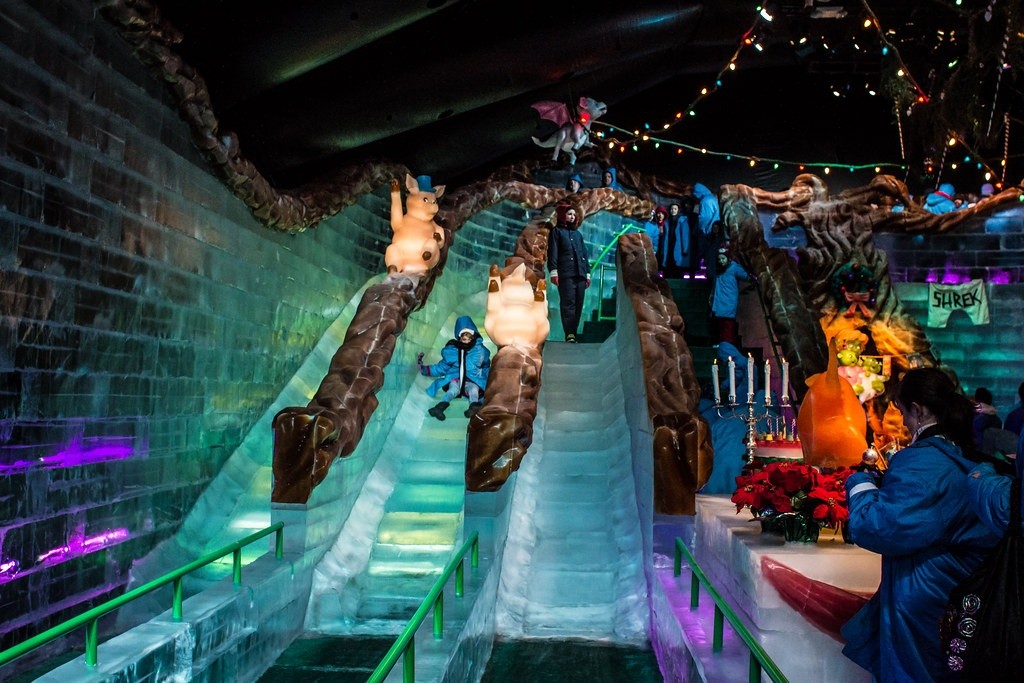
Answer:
[762, 510, 785, 536]
[783, 489, 821, 543]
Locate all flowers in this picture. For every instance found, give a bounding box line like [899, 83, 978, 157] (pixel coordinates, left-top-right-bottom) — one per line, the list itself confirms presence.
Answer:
[731, 460, 786, 520]
[771, 460, 852, 535]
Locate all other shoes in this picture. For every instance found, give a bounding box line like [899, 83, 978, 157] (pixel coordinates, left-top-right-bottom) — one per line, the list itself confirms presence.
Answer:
[566, 334, 576, 343]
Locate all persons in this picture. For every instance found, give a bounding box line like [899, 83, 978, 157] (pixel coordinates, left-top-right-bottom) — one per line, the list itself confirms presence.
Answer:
[839, 364, 1024, 682]
[567, 167, 724, 281]
[921, 184, 969, 215]
[709, 242, 759, 409]
[548, 203, 592, 346]
[416, 314, 494, 423]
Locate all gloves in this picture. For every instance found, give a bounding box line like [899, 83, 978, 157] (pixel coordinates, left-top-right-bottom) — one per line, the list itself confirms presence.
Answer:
[550, 270, 559, 285]
[417, 352, 425, 370]
[749, 275, 760, 286]
[584, 273, 590, 288]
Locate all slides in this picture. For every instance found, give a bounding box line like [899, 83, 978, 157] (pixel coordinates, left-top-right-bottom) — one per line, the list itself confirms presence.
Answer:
[301, 291, 519, 644]
[116, 264, 417, 640]
[500, 332, 654, 650]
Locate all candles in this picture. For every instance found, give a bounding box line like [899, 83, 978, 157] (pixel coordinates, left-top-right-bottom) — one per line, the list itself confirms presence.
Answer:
[781, 357, 789, 401]
[764, 359, 771, 403]
[727, 355, 736, 397]
[712, 358, 721, 400]
[747, 352, 755, 396]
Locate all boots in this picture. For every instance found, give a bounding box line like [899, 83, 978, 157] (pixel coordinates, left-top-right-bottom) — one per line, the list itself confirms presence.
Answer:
[464, 402, 484, 417]
[429, 402, 449, 420]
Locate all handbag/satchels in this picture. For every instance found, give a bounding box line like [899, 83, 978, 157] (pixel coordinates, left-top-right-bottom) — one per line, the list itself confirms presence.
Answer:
[938, 477, 1024, 683]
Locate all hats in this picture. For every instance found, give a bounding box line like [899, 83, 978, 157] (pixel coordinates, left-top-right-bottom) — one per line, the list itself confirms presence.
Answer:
[717, 245, 729, 258]
